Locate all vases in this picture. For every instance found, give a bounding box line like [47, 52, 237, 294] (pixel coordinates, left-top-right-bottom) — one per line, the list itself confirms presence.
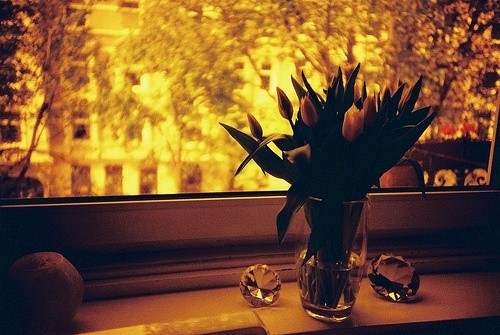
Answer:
[293, 195, 373, 325]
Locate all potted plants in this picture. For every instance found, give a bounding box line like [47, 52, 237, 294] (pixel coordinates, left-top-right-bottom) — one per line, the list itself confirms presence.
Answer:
[380, 142, 424, 184]
[0, 250, 85, 335]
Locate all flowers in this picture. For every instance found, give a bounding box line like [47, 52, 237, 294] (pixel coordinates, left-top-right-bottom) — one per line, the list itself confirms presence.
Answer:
[216, 61, 439, 269]
